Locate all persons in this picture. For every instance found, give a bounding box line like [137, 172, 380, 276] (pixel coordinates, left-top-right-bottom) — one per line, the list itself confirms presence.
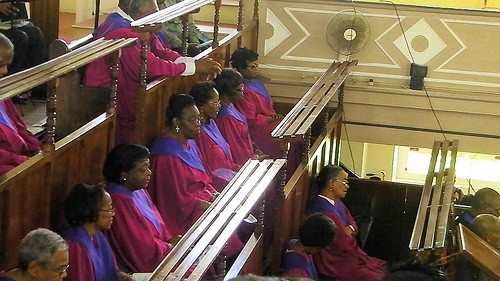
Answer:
[0, 0, 500, 281]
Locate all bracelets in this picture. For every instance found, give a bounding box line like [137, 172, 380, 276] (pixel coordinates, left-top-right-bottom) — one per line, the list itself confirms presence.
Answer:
[348, 225, 356, 232]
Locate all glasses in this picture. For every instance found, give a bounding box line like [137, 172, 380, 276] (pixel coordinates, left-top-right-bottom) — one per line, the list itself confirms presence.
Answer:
[330, 178, 348, 185]
[203, 99, 221, 107]
[183, 115, 203, 121]
[97, 206, 116, 214]
[38, 263, 69, 276]
[231, 84, 245, 94]
[246, 63, 258, 69]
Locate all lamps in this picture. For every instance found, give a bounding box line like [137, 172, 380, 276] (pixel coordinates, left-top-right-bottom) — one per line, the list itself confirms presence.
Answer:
[409, 62, 429, 91]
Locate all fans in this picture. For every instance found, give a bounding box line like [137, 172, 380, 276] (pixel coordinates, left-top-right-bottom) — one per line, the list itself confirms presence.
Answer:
[324, 8, 373, 64]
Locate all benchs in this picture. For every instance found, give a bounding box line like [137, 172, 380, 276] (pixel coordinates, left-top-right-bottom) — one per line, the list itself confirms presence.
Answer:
[25, 123, 48, 145]
[47, 33, 111, 142]
